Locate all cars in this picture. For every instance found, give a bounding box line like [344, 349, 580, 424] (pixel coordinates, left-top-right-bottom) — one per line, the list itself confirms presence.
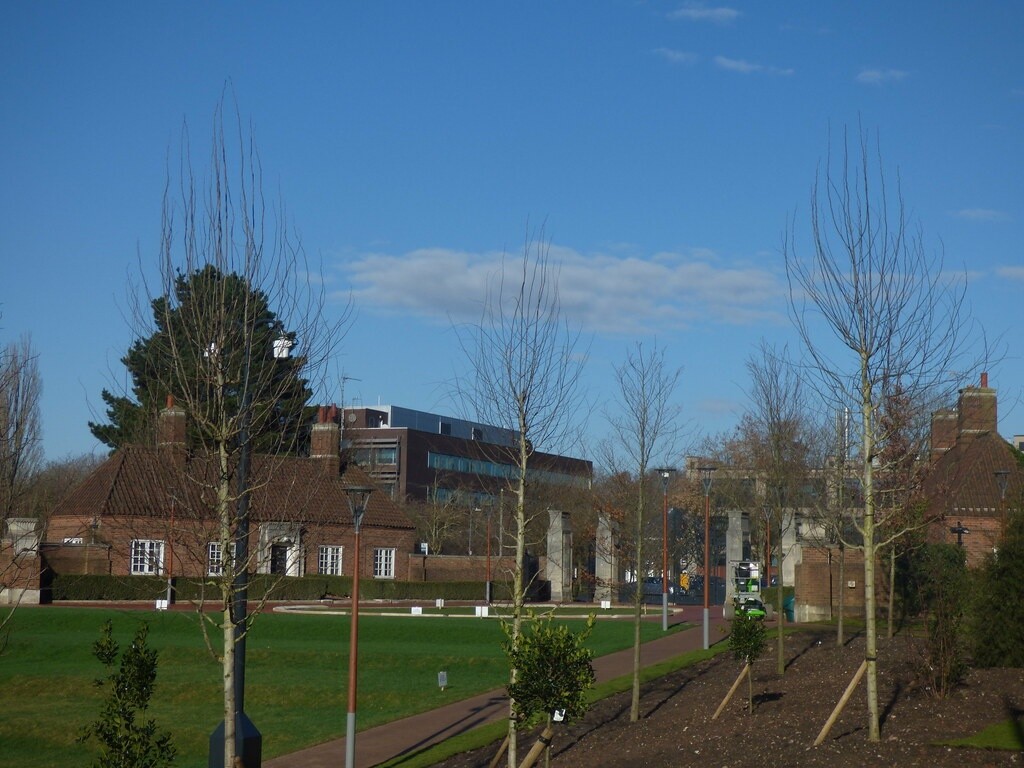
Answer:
[688, 576, 726, 598]
[629, 577, 687, 596]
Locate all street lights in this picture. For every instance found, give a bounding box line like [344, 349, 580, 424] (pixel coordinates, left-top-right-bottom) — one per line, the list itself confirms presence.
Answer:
[485, 504, 494, 603]
[654, 468, 676, 630]
[341, 487, 376, 768]
[696, 467, 718, 650]
[204, 330, 293, 768]
[763, 504, 775, 622]
[166, 487, 178, 605]
